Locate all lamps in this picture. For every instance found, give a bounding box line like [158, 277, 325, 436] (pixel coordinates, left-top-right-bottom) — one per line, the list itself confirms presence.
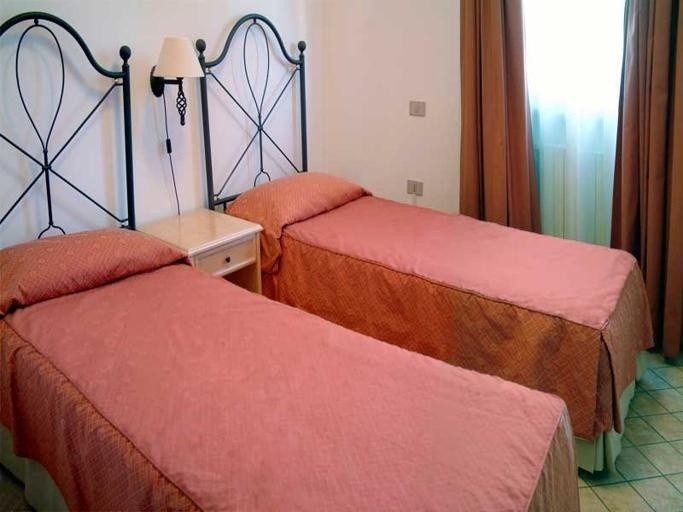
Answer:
[146, 29, 204, 132]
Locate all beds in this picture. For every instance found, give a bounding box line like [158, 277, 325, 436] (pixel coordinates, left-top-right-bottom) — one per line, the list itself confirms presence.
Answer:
[0, 8, 584, 511]
[194, 12, 656, 480]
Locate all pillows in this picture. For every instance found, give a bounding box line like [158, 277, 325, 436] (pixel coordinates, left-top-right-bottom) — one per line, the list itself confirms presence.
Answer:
[0, 228, 185, 318]
[227, 171, 370, 235]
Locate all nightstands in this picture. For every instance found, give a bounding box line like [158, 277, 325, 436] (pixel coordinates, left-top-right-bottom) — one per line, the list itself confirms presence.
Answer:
[137, 205, 265, 295]
